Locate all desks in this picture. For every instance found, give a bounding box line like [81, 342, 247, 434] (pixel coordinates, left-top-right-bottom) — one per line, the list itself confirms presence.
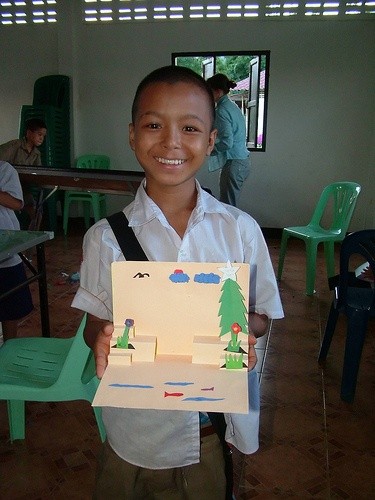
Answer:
[0, 229, 54, 339]
[13, 164, 145, 260]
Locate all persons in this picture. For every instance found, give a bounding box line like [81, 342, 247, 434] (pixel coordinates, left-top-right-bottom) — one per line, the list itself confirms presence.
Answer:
[0, 161, 36, 341]
[0, 117, 46, 219]
[70, 66, 286, 500]
[207, 74, 252, 208]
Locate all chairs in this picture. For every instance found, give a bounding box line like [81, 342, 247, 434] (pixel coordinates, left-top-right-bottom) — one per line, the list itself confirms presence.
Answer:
[64, 154, 111, 238]
[277, 182, 362, 297]
[20, 75, 71, 231]
[318, 229, 375, 404]
[0, 312, 107, 444]
